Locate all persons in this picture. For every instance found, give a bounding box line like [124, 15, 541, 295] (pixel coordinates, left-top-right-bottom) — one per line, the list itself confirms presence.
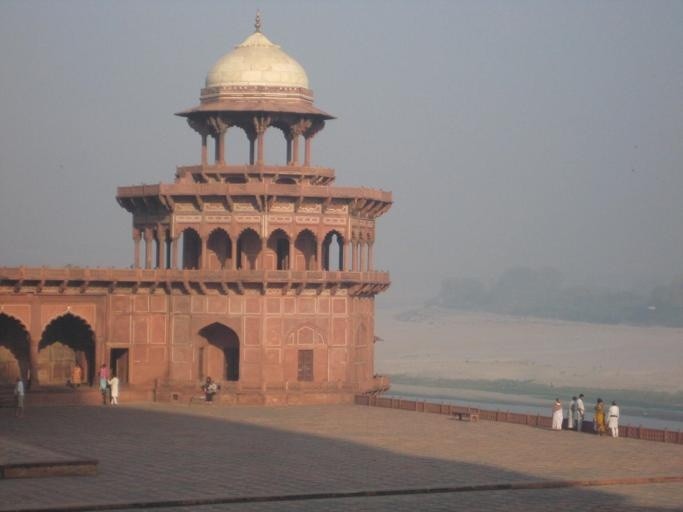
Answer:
[202, 377, 213, 402]
[107, 374, 119, 405]
[98, 364, 108, 392]
[72, 362, 82, 390]
[552, 394, 620, 437]
[207, 380, 216, 393]
[14, 376, 24, 419]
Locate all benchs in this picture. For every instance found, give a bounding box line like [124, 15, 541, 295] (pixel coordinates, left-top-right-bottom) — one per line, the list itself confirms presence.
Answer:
[453, 411, 480, 422]
